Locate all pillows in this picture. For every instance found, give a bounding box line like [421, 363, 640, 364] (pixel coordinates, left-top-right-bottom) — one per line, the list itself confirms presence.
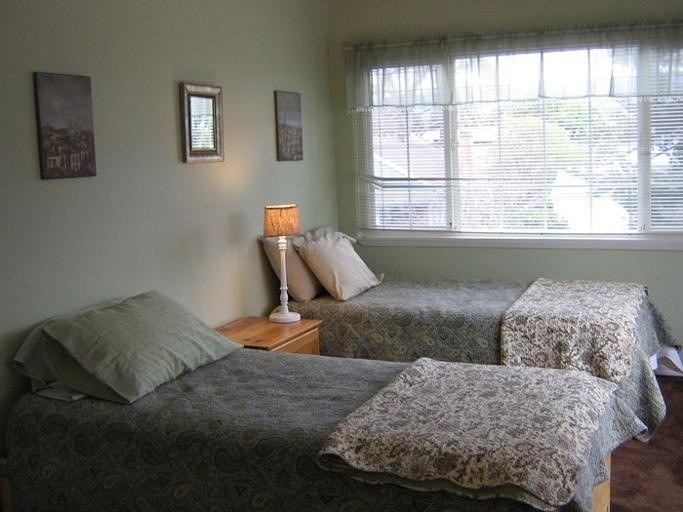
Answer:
[258, 225, 390, 303]
[10, 284, 241, 408]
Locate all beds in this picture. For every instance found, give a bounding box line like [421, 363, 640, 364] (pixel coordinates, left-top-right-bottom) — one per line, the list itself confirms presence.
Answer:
[274, 275, 649, 434]
[2, 346, 614, 511]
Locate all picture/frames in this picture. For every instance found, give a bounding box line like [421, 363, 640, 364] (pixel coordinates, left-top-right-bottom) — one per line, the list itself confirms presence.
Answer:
[179, 81, 224, 164]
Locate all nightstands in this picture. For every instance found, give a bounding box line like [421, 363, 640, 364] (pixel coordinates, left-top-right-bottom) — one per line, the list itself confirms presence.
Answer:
[210, 315, 325, 357]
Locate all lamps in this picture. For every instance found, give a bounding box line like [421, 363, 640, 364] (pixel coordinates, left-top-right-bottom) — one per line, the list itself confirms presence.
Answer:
[263, 203, 301, 325]
